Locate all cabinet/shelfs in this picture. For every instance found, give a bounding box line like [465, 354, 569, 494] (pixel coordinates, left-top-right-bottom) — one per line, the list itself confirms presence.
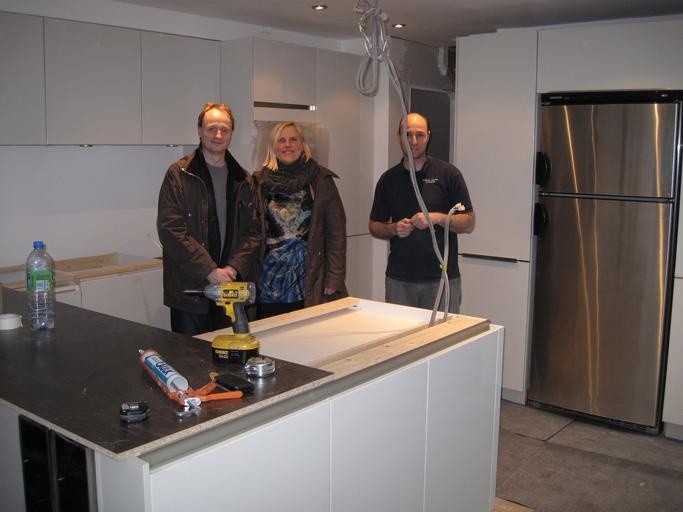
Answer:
[226, 29, 374, 239]
[431, 29, 536, 407]
[0, 10, 223, 149]
[541, 14, 683, 101]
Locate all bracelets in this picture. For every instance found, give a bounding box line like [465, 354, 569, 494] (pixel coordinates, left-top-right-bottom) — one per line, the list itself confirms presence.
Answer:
[390, 222, 397, 236]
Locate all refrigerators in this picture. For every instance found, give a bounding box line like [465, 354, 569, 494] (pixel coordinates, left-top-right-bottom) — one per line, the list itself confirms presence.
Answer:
[525, 90, 683, 428]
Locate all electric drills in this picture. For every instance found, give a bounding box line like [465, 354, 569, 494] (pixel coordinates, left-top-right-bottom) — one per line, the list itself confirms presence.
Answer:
[181, 282, 261, 365]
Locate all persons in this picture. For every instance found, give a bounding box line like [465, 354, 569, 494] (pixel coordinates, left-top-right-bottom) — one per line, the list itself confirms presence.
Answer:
[252, 121, 350, 321]
[156, 102, 267, 336]
[367, 111, 477, 314]
